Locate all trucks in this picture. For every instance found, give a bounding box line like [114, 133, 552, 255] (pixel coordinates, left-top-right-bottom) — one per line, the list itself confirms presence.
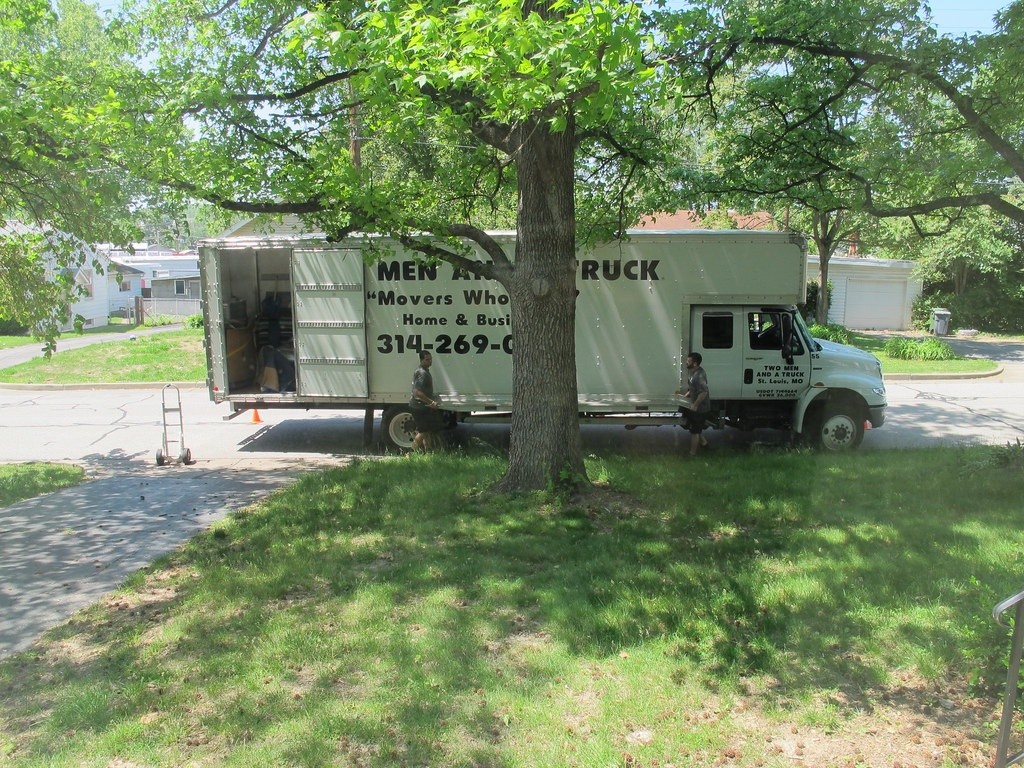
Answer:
[196, 229, 888, 455]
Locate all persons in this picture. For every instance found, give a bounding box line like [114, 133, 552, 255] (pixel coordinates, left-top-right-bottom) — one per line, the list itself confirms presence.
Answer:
[408, 350, 442, 455]
[682, 352, 712, 457]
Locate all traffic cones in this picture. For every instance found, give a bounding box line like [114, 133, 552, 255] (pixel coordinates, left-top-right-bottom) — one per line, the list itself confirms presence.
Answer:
[863, 421, 871, 430]
[251, 408, 264, 424]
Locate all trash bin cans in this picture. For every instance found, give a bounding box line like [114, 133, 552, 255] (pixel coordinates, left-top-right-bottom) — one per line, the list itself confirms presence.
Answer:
[926, 308, 947, 334]
[934, 311, 951, 337]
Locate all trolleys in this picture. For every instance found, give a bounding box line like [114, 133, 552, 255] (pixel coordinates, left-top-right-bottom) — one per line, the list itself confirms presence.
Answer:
[156, 383, 191, 466]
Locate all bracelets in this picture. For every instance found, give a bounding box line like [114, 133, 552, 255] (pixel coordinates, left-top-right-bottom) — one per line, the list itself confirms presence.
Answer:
[430, 400, 435, 406]
